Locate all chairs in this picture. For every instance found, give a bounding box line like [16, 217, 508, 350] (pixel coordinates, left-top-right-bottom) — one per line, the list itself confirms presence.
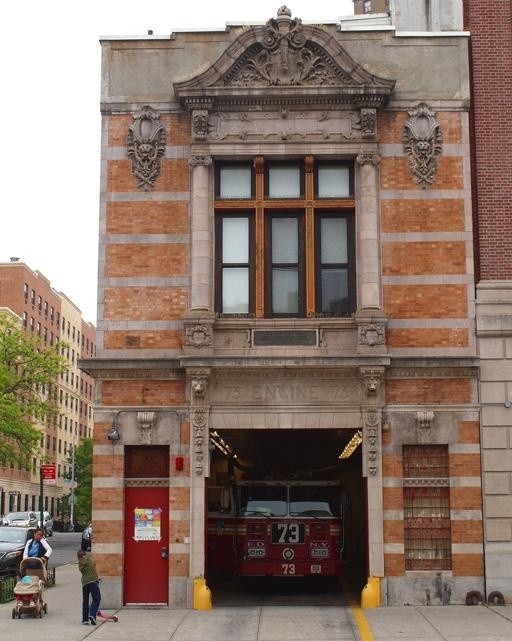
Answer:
[81, 620, 88, 625]
[89, 615, 96, 625]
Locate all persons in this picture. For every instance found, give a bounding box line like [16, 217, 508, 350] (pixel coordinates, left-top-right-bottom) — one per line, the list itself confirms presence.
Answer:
[77, 550, 101, 625]
[22, 529, 52, 566]
[16, 571, 35, 609]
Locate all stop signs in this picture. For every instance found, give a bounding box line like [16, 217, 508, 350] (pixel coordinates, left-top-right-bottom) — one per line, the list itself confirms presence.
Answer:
[206, 466, 352, 594]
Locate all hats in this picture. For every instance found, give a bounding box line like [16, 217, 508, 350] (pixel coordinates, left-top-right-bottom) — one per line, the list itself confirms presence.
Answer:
[0, 523, 48, 577]
[81, 524, 92, 552]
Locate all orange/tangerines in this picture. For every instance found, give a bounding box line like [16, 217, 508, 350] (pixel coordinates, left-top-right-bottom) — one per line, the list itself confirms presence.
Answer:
[12, 557, 48, 620]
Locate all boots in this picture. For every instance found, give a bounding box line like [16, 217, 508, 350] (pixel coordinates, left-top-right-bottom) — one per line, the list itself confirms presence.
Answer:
[0, 509, 54, 536]
